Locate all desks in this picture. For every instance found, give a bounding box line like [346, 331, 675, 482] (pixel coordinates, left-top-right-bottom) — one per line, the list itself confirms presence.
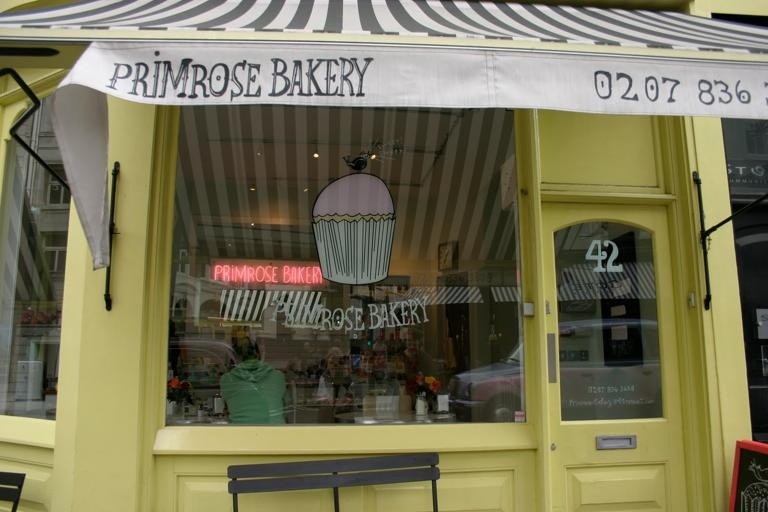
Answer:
[334, 412, 456, 423]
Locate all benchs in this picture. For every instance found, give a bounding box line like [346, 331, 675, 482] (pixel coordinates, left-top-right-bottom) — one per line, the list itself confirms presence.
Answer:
[0, 471, 26, 511]
[227, 452, 441, 512]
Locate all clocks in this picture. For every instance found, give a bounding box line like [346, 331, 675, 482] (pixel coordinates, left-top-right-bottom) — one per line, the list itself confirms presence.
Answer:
[438, 240, 459, 272]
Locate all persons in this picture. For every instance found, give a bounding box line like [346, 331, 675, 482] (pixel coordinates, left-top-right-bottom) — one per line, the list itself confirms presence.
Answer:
[219, 336, 287, 425]
[316, 347, 353, 400]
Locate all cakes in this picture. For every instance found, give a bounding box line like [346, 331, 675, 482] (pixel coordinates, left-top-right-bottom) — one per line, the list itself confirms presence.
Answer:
[312, 172, 396, 286]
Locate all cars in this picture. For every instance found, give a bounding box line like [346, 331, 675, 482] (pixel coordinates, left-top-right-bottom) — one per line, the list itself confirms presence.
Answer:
[165, 340, 269, 425]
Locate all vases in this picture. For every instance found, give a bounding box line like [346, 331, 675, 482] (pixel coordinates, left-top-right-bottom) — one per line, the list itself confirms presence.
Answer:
[415, 395, 429, 416]
[171, 399, 185, 420]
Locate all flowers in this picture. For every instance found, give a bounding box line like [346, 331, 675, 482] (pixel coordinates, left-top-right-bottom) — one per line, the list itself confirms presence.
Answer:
[167, 375, 195, 406]
[415, 374, 443, 402]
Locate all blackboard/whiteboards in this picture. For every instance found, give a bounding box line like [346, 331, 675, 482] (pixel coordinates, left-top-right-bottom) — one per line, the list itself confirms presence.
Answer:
[729, 439, 768, 512]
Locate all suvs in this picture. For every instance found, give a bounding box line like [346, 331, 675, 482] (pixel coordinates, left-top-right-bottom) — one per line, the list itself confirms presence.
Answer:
[446, 318, 662, 422]
